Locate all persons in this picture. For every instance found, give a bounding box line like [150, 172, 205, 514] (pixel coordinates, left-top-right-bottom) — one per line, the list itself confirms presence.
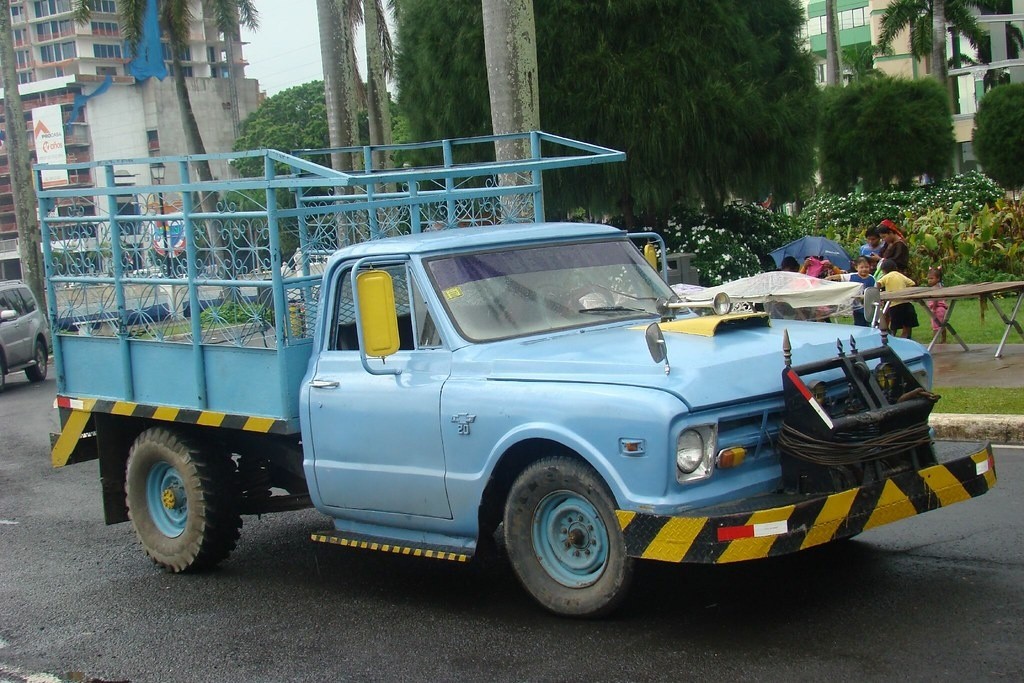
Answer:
[763, 224, 917, 339]
[927, 269, 948, 344]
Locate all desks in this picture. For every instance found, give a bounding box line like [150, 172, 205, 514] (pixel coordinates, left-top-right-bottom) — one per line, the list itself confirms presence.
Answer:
[851, 281, 1024, 358]
[581, 269, 864, 321]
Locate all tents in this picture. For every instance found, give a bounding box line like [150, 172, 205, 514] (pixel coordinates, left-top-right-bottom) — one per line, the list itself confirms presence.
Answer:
[767, 235, 856, 271]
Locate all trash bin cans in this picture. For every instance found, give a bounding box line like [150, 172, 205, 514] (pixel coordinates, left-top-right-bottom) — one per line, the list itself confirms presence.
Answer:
[659, 252, 699, 286]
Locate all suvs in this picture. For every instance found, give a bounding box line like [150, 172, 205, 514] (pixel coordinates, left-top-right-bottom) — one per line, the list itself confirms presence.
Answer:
[0, 279, 51, 392]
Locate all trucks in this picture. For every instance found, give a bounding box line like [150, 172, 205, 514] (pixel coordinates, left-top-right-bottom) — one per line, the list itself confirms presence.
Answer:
[29, 130, 998, 622]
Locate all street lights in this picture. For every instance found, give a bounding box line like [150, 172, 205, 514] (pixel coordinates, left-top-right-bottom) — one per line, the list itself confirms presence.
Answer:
[150, 163, 173, 279]
